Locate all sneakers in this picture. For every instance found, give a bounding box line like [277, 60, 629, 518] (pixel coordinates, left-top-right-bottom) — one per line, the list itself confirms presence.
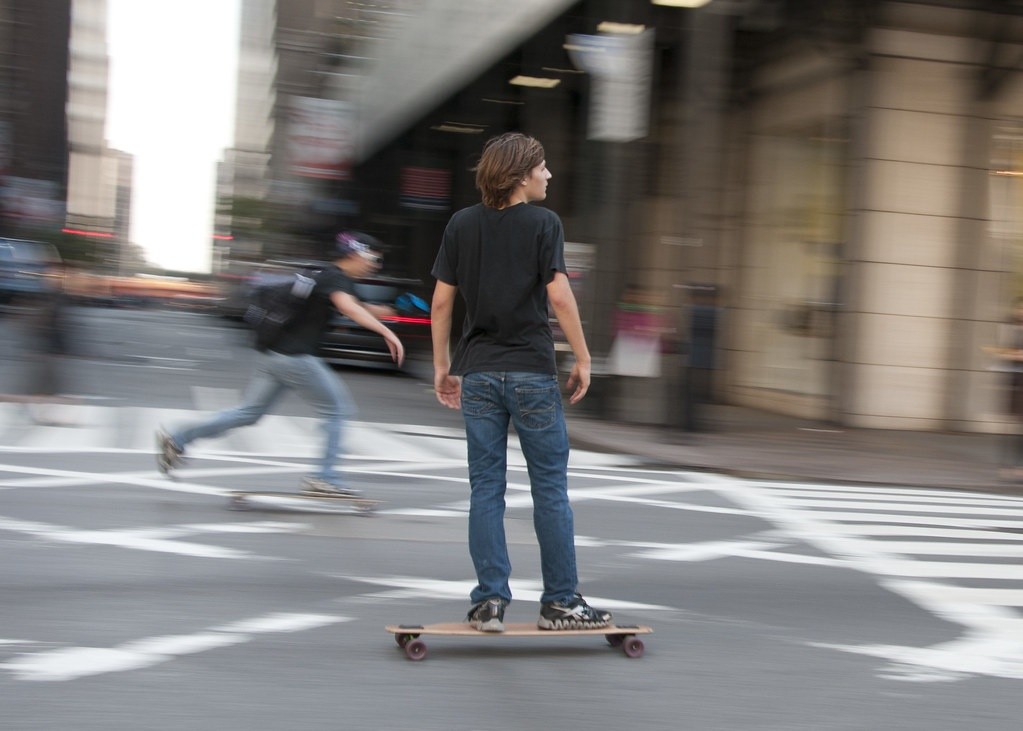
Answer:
[538, 592, 613, 631]
[469, 598, 504, 632]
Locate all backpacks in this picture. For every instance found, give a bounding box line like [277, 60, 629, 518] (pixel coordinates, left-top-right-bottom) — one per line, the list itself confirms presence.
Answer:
[244, 270, 340, 334]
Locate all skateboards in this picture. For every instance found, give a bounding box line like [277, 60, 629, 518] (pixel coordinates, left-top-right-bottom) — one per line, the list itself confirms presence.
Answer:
[223, 488, 385, 514]
[384, 620, 655, 658]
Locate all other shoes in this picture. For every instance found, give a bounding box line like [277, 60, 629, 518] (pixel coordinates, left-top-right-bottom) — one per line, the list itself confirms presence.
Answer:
[303, 475, 362, 498]
[157, 434, 180, 475]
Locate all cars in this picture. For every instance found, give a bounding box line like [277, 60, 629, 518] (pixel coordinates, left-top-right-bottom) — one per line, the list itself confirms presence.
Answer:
[246, 257, 435, 370]
[1, 237, 72, 307]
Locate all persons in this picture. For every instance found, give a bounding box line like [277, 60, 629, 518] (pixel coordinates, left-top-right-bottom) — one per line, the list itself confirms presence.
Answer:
[155, 230, 404, 498]
[431, 133, 612, 631]
